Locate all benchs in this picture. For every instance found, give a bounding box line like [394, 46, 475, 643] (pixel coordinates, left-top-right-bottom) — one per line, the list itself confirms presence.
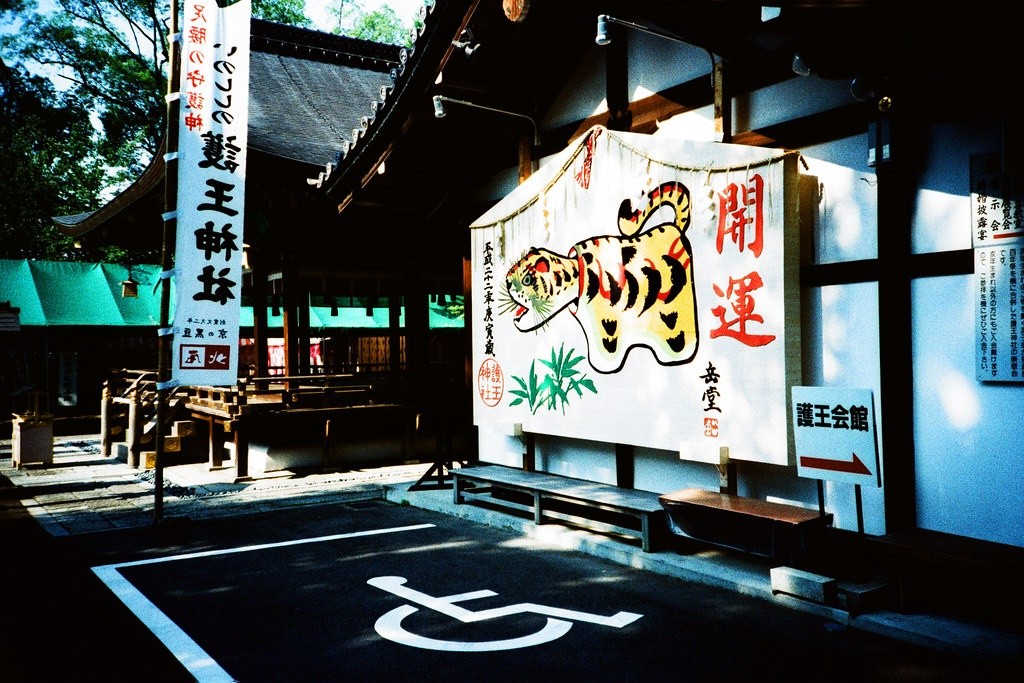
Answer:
[658, 489, 834, 570]
[448, 466, 666, 553]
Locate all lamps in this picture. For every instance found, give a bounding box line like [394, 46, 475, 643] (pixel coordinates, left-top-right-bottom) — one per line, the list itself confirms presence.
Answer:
[432, 95, 541, 147]
[119, 271, 140, 299]
[595, 14, 716, 87]
